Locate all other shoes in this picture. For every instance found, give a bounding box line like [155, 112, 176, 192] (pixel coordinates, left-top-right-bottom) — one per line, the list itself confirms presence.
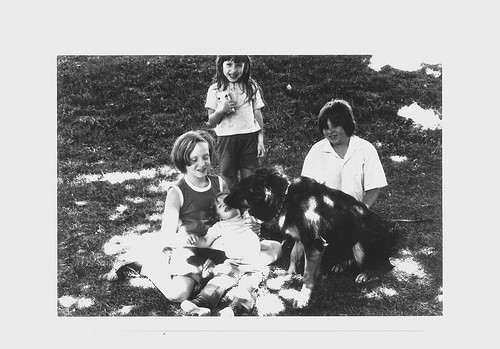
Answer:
[180, 298, 211, 316]
[221, 302, 242, 317]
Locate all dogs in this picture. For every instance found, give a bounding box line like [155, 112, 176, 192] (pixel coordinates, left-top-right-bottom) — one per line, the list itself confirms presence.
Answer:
[224, 167, 396, 309]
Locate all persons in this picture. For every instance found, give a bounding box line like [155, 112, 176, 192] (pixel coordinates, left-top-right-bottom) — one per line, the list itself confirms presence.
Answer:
[107, 130, 282, 302]
[181, 192, 261, 317]
[205, 55, 265, 184]
[300, 100, 388, 210]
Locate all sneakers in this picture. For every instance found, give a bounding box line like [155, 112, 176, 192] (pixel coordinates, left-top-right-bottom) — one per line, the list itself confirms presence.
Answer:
[107, 255, 156, 281]
